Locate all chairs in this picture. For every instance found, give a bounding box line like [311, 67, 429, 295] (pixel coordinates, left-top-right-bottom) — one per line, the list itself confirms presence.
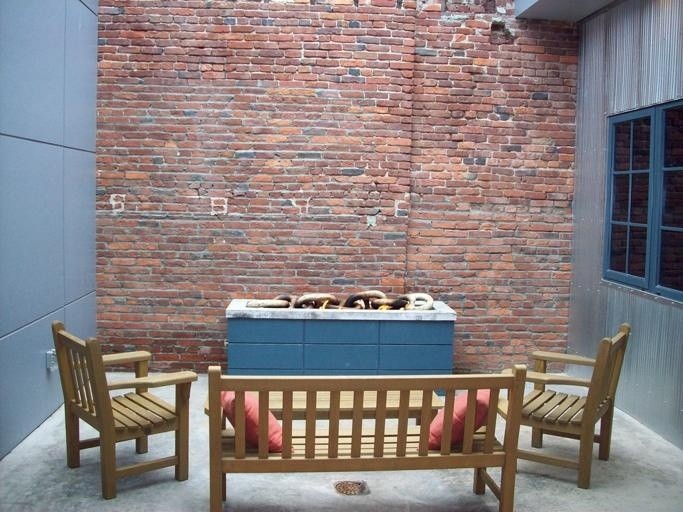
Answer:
[53, 321, 198, 500]
[499, 323, 631, 489]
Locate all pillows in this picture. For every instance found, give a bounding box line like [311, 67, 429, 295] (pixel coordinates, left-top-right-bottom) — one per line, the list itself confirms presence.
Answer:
[428, 389, 490, 449]
[220, 391, 282, 452]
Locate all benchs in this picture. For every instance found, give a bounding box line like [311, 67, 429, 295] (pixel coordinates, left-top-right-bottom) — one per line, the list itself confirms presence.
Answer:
[205, 365, 527, 511]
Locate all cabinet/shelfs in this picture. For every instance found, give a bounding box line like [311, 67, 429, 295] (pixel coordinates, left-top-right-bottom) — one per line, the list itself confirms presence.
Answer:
[226, 289, 456, 374]
